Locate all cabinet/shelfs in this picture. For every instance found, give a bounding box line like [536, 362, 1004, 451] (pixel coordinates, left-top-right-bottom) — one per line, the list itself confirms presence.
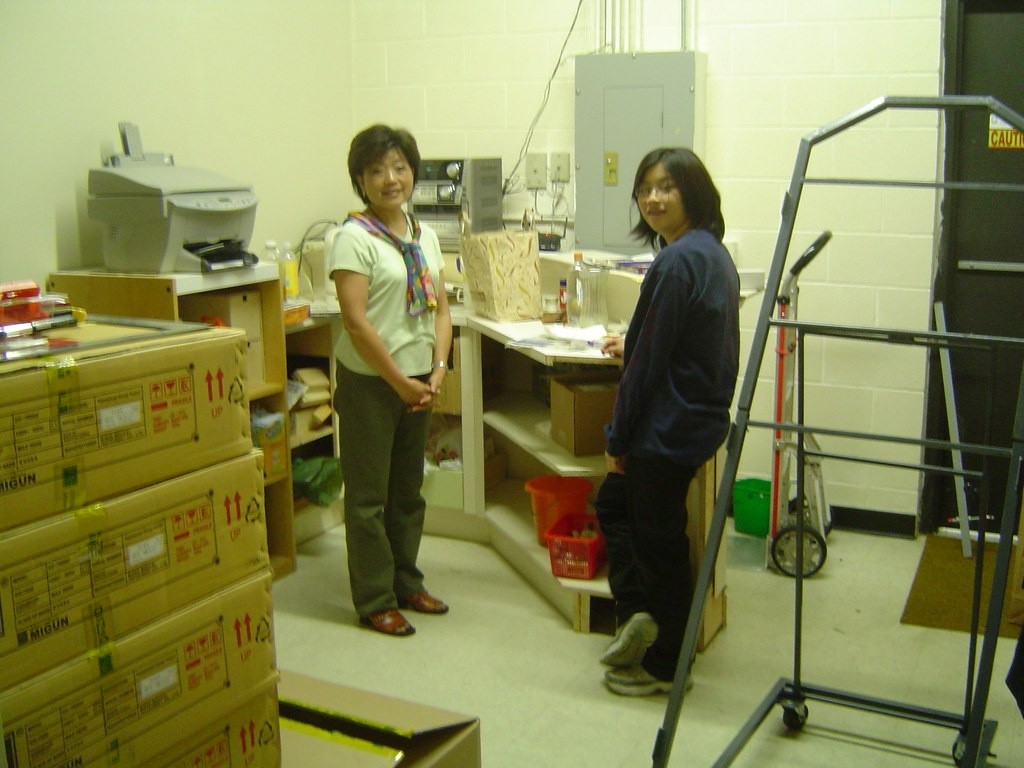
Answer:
[46, 262, 295, 581]
[468, 314, 623, 634]
[286, 317, 345, 547]
[421, 295, 468, 543]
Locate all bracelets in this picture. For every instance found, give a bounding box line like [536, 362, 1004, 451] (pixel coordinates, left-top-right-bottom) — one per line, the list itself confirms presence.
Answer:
[432, 361, 448, 373]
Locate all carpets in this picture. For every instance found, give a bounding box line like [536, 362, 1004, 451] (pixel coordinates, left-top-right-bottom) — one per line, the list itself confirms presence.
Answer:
[899, 533, 1023, 642]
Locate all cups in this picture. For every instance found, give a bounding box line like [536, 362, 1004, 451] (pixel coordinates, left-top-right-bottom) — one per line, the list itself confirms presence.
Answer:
[578, 270, 609, 330]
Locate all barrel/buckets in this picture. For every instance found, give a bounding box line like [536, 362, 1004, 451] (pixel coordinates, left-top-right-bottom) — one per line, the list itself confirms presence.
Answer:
[525, 476, 591, 548]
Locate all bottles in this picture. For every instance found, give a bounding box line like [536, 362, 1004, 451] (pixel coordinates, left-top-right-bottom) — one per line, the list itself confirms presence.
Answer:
[279, 242, 299, 305]
[567, 253, 590, 328]
[559, 280, 567, 324]
[263, 241, 280, 262]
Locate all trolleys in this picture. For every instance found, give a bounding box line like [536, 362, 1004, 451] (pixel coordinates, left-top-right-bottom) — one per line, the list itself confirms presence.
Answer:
[764, 228, 836, 581]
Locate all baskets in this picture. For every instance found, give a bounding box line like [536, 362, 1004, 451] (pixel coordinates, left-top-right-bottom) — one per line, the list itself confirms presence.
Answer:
[544, 512, 608, 578]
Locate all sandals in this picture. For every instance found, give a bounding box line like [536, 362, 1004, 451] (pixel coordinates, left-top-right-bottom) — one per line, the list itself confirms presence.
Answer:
[398, 589, 449, 614]
[359, 608, 416, 637]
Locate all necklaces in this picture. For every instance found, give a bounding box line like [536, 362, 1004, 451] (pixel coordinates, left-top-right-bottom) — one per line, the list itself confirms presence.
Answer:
[368, 205, 413, 237]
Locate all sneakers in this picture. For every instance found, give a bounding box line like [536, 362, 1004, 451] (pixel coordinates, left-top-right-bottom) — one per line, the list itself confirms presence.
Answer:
[599, 612, 693, 695]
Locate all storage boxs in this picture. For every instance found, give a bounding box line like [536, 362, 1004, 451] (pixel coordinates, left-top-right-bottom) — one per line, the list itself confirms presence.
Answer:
[0, 290, 624, 768]
[732, 479, 771, 538]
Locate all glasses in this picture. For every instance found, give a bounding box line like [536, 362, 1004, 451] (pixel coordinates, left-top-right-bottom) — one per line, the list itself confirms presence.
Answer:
[633, 181, 675, 197]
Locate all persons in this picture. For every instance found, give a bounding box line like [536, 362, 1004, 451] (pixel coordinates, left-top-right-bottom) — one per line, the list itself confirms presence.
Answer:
[328, 124, 452, 637]
[598, 147, 741, 695]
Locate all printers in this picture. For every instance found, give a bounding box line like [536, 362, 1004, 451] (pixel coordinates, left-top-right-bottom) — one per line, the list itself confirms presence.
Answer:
[87, 153, 259, 275]
